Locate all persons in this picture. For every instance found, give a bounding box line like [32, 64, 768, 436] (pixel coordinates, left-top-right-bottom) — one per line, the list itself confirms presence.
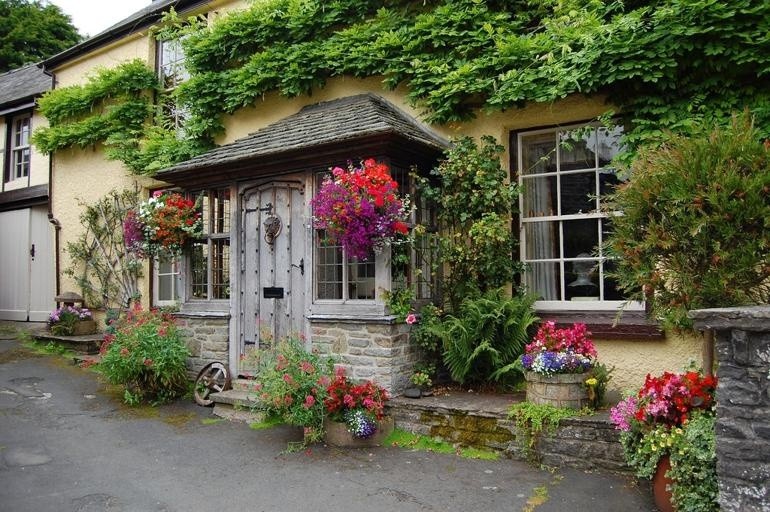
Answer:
[566, 250, 600, 301]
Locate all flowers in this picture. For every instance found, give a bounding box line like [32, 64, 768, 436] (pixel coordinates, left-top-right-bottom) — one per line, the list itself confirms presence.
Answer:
[234, 326, 394, 455]
[45, 304, 92, 335]
[76, 303, 192, 409]
[303, 158, 417, 264]
[609, 357, 721, 511]
[124, 189, 208, 266]
[520, 318, 602, 379]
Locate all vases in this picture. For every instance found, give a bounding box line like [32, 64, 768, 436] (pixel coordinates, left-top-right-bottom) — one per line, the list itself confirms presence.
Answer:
[526, 368, 597, 414]
[651, 452, 693, 511]
[136, 369, 165, 402]
[320, 416, 387, 448]
[71, 320, 97, 335]
